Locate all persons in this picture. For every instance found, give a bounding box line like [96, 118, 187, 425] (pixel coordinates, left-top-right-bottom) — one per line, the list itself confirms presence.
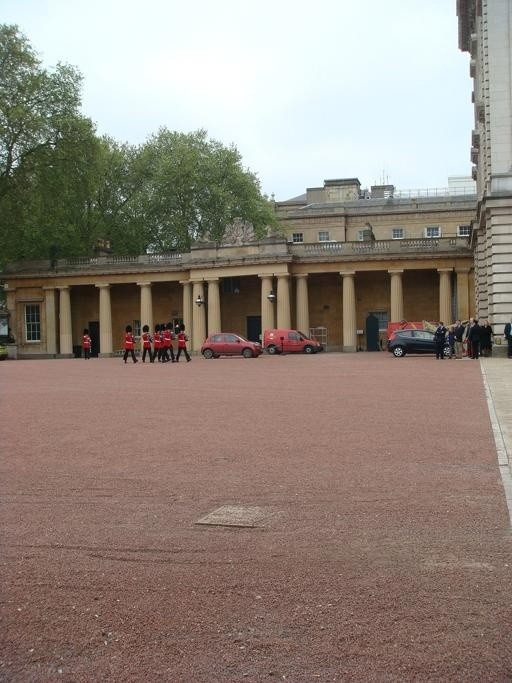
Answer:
[504, 320, 512, 358]
[123, 323, 190, 363]
[82, 327, 92, 359]
[434, 317, 492, 362]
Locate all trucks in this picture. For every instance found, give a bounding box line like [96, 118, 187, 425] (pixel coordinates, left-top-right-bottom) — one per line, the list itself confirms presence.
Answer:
[387, 320, 444, 347]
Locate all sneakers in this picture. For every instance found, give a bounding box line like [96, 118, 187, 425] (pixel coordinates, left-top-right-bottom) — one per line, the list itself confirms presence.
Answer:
[142, 358, 192, 362]
[134, 360, 138, 363]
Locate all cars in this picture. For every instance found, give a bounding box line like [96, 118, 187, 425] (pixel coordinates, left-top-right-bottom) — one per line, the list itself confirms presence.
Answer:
[388, 328, 454, 356]
[201, 331, 264, 358]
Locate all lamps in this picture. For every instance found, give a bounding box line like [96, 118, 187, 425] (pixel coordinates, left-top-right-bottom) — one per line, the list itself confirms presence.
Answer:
[267, 290, 277, 305]
[194, 294, 206, 307]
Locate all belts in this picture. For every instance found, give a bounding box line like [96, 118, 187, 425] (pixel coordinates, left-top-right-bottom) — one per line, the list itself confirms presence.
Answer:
[156, 340, 161, 342]
[126, 341, 132, 343]
[165, 339, 170, 340]
[179, 340, 184, 341]
[144, 341, 149, 342]
[84, 342, 90, 343]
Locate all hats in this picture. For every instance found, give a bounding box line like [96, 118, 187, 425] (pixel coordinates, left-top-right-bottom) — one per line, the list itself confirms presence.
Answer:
[143, 325, 148, 332]
[180, 324, 185, 331]
[155, 323, 173, 332]
[126, 325, 132, 332]
[83, 329, 88, 334]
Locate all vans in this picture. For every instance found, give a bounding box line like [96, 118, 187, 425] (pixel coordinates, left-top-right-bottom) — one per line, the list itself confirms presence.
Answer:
[264, 328, 324, 352]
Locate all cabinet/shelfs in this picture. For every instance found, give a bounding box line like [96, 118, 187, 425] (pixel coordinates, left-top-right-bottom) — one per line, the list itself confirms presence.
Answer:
[309, 327, 328, 353]
